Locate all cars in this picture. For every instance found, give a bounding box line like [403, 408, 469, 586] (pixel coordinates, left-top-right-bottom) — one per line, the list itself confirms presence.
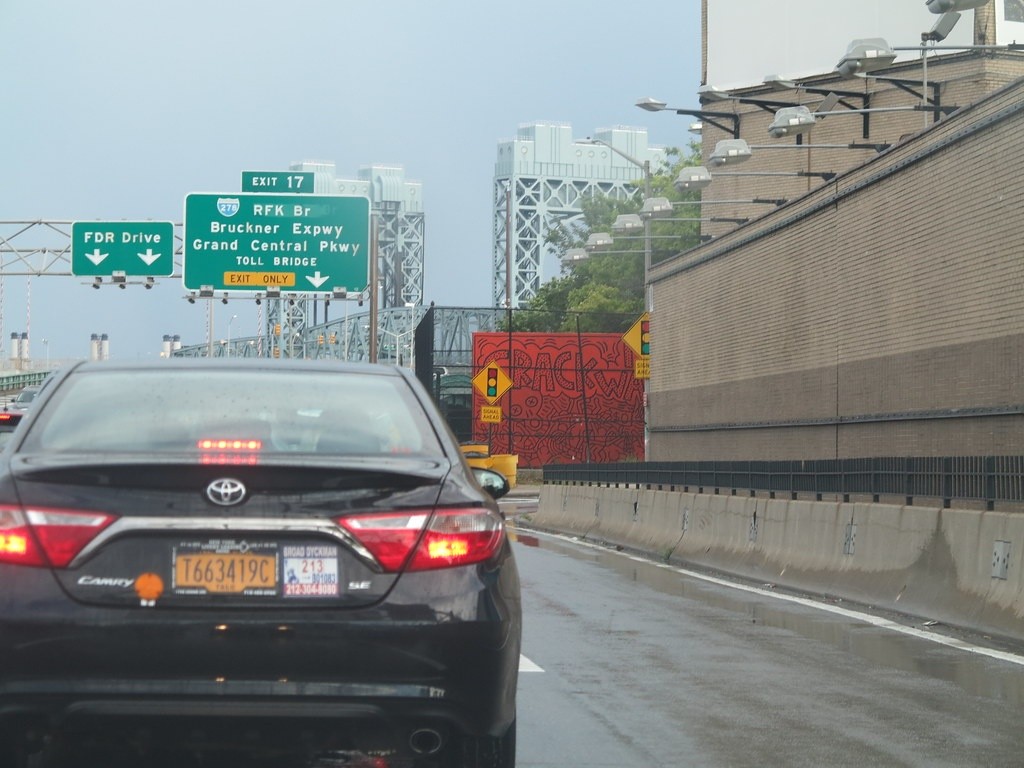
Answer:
[0, 357, 523, 768]
[10, 385, 41, 408]
[0, 404, 29, 454]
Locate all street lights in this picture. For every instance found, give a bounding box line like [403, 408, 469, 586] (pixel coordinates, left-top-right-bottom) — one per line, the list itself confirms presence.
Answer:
[575, 138, 651, 462]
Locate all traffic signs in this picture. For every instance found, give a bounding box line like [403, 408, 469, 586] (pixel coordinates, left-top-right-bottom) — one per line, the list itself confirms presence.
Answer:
[70, 220, 175, 278]
[181, 192, 371, 294]
